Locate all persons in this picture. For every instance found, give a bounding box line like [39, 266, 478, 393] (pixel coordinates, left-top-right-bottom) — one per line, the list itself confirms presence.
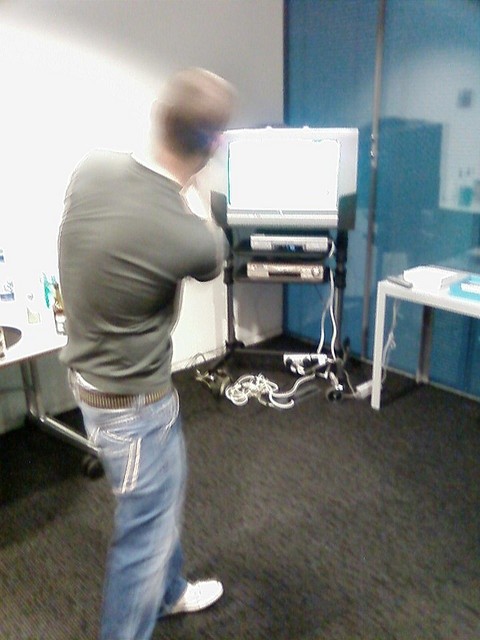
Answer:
[57, 68, 231, 640]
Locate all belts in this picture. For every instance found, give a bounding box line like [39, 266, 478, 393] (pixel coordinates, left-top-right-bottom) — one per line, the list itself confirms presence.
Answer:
[66, 367, 176, 411]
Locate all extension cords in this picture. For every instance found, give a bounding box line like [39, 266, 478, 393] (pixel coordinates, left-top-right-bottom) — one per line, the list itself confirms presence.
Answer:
[282, 353, 327, 370]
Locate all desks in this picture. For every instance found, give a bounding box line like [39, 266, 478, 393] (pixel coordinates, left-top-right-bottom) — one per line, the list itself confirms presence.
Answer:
[0, 322, 102, 479]
[371, 265, 480, 410]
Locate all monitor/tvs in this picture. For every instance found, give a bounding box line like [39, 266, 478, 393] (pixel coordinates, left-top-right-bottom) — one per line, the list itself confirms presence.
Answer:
[221, 126, 359, 231]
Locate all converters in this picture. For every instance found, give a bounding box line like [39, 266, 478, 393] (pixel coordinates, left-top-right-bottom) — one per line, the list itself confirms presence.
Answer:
[356, 379, 383, 399]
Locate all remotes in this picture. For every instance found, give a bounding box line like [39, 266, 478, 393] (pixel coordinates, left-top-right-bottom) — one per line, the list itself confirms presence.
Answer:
[387, 274, 414, 289]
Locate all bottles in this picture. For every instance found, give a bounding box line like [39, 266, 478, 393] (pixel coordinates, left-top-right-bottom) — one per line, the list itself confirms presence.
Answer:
[52, 283, 71, 336]
[39, 272, 52, 311]
[0, 247, 15, 303]
[26, 294, 41, 323]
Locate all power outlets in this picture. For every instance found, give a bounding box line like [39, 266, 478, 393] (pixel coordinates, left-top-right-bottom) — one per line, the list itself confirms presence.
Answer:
[283, 354, 327, 367]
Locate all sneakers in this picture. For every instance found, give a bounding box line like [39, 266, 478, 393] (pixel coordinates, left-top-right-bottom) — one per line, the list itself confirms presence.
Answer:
[157, 577, 224, 617]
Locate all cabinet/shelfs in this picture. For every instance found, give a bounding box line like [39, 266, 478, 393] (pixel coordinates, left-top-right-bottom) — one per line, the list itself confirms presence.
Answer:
[202, 225, 348, 401]
